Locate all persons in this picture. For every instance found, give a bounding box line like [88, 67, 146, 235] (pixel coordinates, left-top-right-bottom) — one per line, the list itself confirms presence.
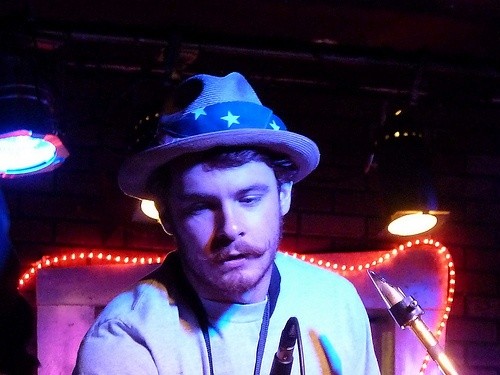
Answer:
[72, 72, 381, 375]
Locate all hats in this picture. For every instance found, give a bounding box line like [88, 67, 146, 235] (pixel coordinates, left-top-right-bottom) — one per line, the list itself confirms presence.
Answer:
[118, 71, 321, 201]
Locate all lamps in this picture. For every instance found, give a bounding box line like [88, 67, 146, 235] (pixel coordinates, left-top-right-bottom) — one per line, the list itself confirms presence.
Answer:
[0, 95, 69, 178]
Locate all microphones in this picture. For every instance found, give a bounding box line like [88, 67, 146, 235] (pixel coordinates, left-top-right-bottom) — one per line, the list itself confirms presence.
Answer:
[269, 317, 299, 375]
